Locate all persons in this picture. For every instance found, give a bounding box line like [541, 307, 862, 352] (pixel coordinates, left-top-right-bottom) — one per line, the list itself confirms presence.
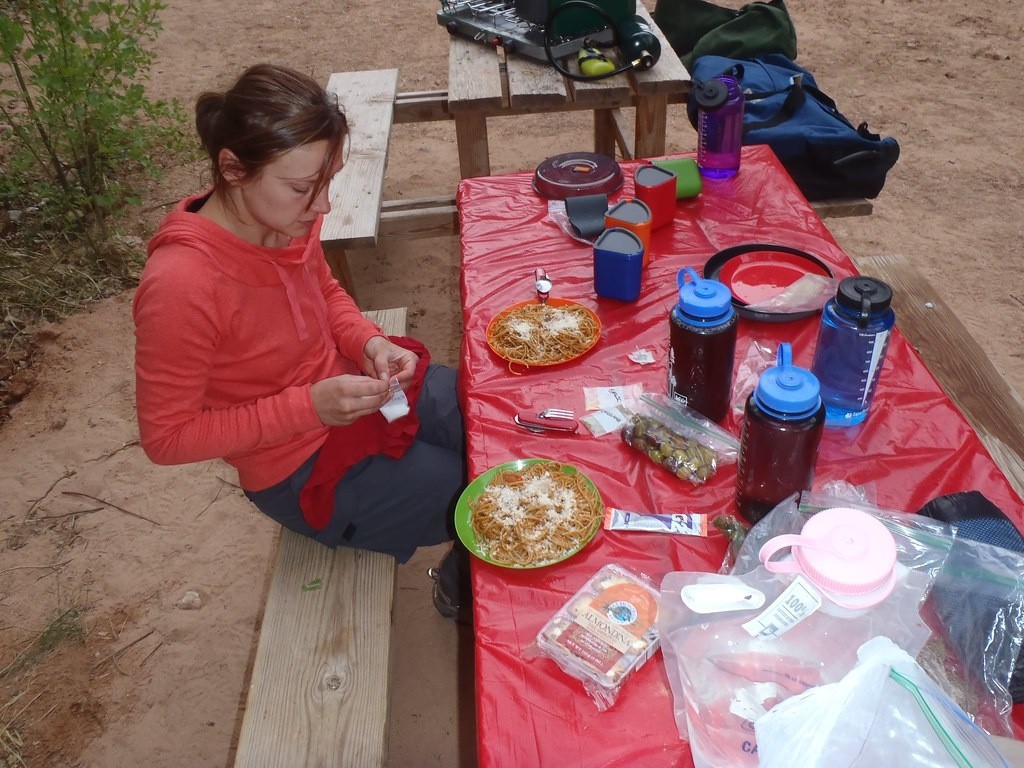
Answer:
[132, 62, 477, 617]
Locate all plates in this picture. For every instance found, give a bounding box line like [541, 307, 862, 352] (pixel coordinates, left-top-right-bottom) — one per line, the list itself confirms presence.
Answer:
[485, 297, 603, 366]
[454, 458, 604, 569]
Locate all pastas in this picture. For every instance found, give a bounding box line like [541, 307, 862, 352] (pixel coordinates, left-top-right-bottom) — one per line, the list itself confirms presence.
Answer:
[489, 303, 601, 375]
[469, 460, 605, 566]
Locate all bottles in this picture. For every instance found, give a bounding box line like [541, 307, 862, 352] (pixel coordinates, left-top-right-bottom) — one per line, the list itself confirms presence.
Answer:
[668, 266, 741, 423]
[695, 75, 746, 180]
[808, 275, 896, 429]
[756, 506, 897, 681]
[734, 341, 827, 527]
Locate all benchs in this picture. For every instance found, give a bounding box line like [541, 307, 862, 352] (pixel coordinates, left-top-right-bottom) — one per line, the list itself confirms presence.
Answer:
[853, 251, 1024, 501]
[314, 66, 402, 246]
[809, 197, 876, 219]
[232, 305, 409, 768]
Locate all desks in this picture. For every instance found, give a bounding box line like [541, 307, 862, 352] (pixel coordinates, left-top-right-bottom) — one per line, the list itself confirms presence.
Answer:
[446, 0, 695, 180]
[456, 144, 1024, 768]
